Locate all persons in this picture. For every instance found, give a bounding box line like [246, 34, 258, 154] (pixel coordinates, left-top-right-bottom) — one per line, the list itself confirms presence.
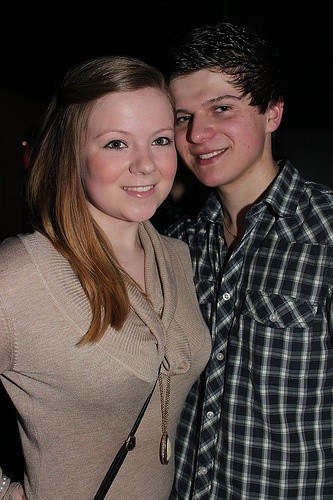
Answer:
[161, 23, 333, 500]
[0, 56, 213, 500]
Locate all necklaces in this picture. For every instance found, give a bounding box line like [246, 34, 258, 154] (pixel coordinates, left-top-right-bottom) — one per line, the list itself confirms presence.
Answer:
[220, 214, 241, 243]
